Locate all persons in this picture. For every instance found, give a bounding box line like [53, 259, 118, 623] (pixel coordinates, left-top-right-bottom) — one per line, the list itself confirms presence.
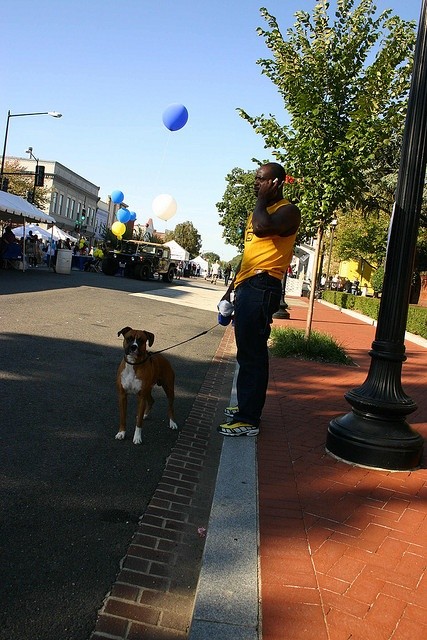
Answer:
[210, 260, 220, 284]
[157, 251, 162, 256]
[2, 226, 15, 238]
[216, 162, 303, 436]
[176, 261, 201, 280]
[224, 263, 231, 286]
[25, 231, 107, 267]
[320, 273, 367, 297]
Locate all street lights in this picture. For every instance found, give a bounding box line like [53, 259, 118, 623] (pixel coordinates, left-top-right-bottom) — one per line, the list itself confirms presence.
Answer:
[0, 110, 62, 191]
[25, 147, 40, 205]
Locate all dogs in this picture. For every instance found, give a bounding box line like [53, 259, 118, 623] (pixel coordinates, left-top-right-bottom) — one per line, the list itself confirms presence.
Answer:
[114, 326, 177, 445]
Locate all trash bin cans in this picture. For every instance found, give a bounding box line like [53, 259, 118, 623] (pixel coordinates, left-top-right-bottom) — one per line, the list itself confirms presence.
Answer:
[56, 249, 72, 274]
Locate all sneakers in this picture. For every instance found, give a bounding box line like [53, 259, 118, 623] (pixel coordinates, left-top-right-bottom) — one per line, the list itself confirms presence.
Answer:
[215, 420, 259, 437]
[223, 405, 239, 418]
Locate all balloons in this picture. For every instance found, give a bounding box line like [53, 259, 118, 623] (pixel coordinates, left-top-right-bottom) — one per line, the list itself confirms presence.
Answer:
[111, 190, 124, 203]
[117, 235, 122, 240]
[111, 221, 126, 236]
[162, 103, 188, 131]
[129, 211, 136, 221]
[152, 194, 177, 222]
[116, 208, 131, 223]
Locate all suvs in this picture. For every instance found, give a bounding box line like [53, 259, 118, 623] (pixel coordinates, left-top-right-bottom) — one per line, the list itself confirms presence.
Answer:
[102, 239, 177, 283]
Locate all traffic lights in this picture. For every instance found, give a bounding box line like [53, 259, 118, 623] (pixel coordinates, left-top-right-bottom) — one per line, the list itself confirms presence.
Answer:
[80, 208, 87, 222]
[82, 224, 89, 236]
[34, 165, 46, 188]
[74, 218, 81, 234]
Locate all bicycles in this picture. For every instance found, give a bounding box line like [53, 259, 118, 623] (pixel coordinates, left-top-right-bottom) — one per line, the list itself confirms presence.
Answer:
[224, 269, 230, 287]
[211, 272, 222, 285]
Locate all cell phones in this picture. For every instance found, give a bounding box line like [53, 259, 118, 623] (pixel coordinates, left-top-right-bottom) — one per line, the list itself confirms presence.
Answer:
[272, 178, 284, 188]
